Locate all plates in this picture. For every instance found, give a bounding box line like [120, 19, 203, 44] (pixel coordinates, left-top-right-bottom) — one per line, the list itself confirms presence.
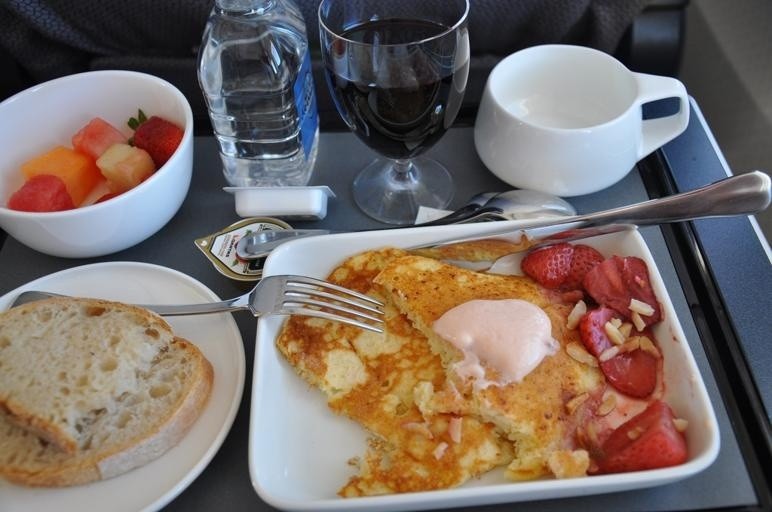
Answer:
[0, 260, 247, 512]
[248, 215, 720, 512]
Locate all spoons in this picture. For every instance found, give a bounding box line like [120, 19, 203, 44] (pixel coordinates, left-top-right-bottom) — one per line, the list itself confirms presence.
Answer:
[236, 190, 576, 258]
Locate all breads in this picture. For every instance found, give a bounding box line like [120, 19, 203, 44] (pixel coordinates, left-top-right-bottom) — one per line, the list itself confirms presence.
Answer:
[0, 295, 214, 490]
[275, 246, 603, 497]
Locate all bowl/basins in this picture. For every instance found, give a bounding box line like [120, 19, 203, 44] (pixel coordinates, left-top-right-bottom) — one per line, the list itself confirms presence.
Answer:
[0, 69, 193, 258]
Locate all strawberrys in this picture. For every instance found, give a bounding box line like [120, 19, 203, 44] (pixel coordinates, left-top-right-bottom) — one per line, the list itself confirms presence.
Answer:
[579, 255, 663, 326]
[520, 244, 573, 289]
[577, 306, 658, 399]
[561, 244, 606, 294]
[126, 109, 183, 171]
[584, 399, 688, 476]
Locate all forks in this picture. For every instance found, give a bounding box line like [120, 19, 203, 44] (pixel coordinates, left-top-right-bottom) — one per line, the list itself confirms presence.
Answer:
[11, 274, 386, 336]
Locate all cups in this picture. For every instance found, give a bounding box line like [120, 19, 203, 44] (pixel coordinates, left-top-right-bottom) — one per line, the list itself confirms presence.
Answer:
[473, 44, 690, 198]
[317, 1, 470, 225]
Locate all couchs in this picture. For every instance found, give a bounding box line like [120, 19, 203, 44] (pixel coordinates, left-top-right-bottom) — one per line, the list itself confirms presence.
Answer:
[2, 0, 688, 129]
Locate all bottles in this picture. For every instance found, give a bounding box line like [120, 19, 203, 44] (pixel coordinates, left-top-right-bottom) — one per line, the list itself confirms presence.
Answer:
[195, 1, 320, 189]
